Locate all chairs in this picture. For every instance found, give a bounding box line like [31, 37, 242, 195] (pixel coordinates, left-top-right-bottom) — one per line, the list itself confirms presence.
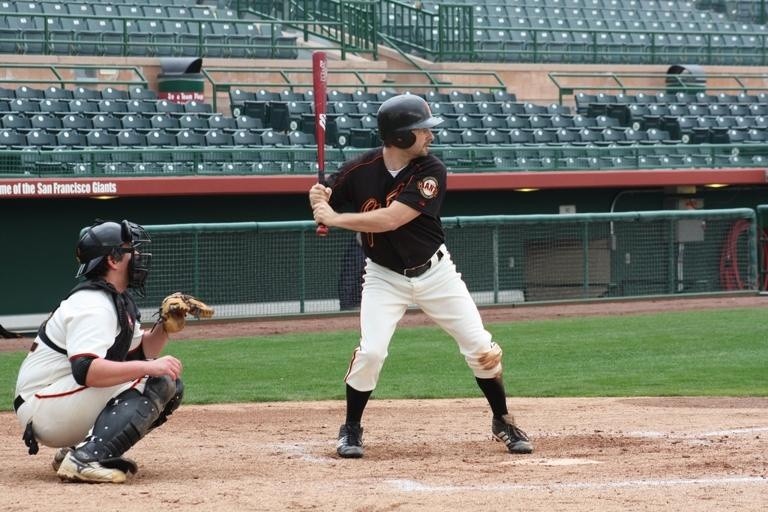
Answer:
[0, 0, 298, 65]
[425, 89, 766, 170]
[382, 3, 764, 64]
[0, 87, 397, 174]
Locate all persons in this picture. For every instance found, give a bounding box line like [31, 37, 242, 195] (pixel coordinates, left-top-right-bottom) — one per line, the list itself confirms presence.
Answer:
[307, 91, 535, 458]
[13, 217, 215, 485]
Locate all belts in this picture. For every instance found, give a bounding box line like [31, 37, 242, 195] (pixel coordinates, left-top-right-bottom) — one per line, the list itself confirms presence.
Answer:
[396, 251, 443, 277]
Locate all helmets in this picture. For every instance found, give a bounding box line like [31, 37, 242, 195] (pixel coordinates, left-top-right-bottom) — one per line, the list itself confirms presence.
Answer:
[378, 94, 445, 148]
[74, 219, 152, 299]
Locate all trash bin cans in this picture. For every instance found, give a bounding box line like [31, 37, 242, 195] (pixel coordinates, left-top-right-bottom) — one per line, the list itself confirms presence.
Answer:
[158, 57, 205, 102]
[666, 63, 706, 96]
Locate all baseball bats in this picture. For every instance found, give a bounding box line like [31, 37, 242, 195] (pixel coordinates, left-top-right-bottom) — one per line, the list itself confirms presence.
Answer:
[313, 50, 328, 236]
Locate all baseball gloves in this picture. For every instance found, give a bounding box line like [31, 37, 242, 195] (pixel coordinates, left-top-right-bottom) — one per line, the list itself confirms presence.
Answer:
[160, 292, 213, 332]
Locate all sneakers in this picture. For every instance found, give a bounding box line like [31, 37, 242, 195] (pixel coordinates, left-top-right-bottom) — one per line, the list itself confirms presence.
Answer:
[51, 448, 138, 483]
[491, 413, 533, 452]
[336, 424, 364, 458]
